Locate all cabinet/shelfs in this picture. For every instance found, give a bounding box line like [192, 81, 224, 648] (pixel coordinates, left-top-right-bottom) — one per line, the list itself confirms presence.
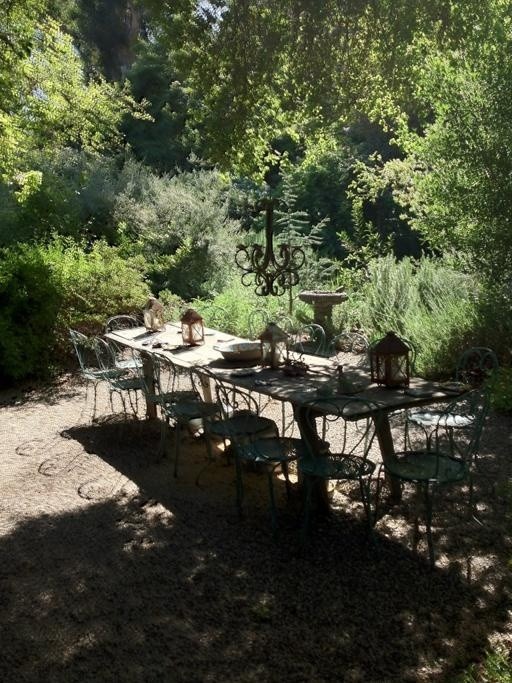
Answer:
[66, 308, 501, 566]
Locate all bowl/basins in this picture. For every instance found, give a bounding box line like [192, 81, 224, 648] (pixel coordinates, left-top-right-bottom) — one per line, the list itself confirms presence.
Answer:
[213, 342, 268, 363]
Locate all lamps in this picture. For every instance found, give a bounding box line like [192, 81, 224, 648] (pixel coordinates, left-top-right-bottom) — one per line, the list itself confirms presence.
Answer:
[141, 297, 165, 332]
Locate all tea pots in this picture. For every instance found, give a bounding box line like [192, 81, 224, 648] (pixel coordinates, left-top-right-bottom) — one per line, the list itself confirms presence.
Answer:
[338, 373, 368, 394]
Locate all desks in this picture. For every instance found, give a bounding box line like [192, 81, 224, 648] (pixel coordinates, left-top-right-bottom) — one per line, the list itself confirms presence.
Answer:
[299, 289, 349, 349]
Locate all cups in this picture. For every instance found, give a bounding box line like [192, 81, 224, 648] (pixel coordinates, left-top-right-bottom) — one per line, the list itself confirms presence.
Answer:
[317, 381, 332, 396]
